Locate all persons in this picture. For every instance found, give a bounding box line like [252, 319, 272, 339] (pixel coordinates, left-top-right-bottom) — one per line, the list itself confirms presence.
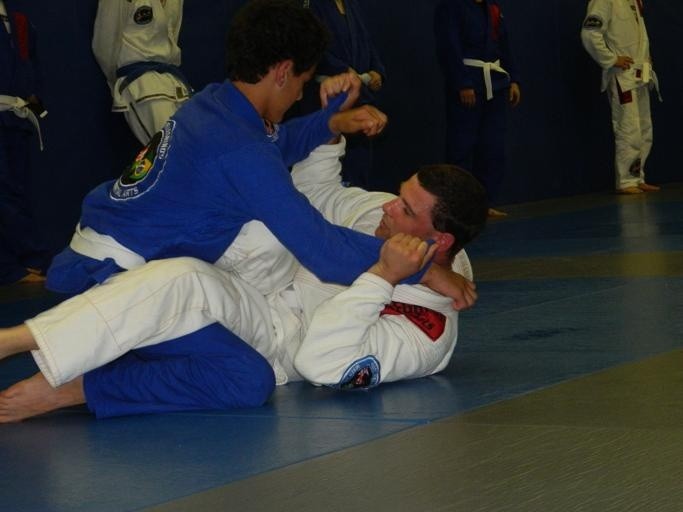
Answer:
[578, 0, 664, 194]
[0, 0, 480, 424]
[0, 0, 52, 284]
[0, 68, 490, 393]
[90, 0, 193, 146]
[434, 0, 523, 216]
[298, 0, 388, 190]
